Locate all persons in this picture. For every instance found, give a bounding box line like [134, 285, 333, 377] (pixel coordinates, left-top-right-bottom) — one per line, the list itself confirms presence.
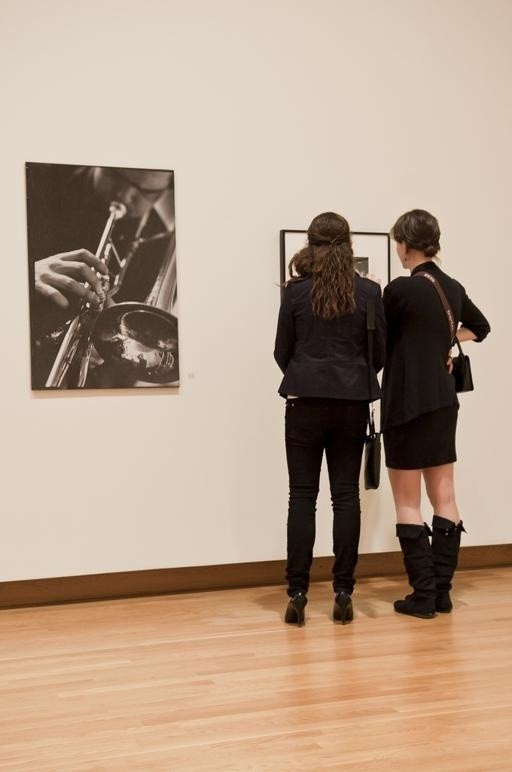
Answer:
[272, 210, 388, 628]
[27, 164, 180, 388]
[379, 210, 490, 619]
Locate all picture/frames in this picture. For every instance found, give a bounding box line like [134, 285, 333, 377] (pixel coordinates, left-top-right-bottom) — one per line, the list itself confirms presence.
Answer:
[280, 229, 391, 298]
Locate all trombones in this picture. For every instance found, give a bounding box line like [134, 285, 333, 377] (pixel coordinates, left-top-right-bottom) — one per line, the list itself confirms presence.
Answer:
[45, 202, 178, 388]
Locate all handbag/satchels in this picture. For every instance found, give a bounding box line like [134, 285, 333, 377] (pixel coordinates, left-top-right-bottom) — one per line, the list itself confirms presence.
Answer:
[452, 356, 473, 392]
[364, 434, 381, 490]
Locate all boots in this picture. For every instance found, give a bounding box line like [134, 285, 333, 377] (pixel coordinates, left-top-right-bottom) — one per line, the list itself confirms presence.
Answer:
[394, 522, 436, 619]
[431, 515, 467, 613]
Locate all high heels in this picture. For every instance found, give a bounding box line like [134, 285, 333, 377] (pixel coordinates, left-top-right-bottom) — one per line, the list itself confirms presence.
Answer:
[333, 592, 353, 625]
[285, 593, 307, 627]
